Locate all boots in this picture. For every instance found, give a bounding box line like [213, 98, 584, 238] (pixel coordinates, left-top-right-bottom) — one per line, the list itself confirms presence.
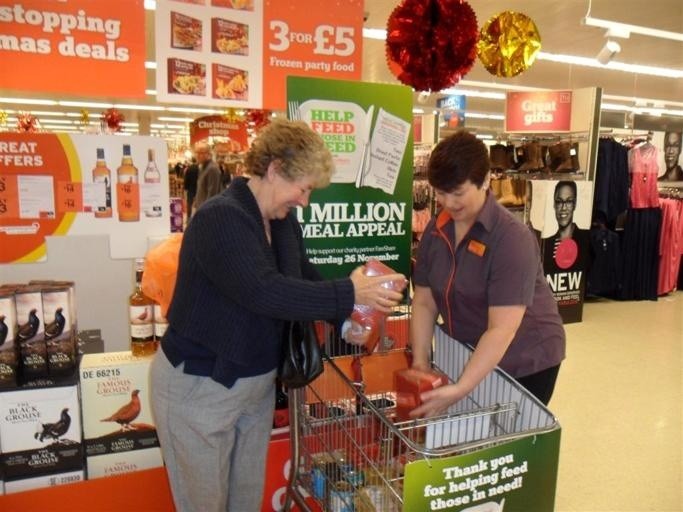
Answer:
[491, 141, 578, 172]
[491, 178, 526, 205]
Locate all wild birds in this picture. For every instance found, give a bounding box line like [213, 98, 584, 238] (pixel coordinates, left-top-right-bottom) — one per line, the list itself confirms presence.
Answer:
[0, 315, 8, 347]
[136, 308, 148, 320]
[16, 309, 40, 347]
[44, 307, 65, 347]
[33, 408, 71, 442]
[99, 390, 141, 432]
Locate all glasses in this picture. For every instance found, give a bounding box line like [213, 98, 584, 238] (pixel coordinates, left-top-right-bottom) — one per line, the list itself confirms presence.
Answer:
[554, 198, 575, 208]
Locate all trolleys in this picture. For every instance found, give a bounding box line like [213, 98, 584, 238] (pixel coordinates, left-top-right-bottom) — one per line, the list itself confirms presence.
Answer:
[282, 256, 561, 511]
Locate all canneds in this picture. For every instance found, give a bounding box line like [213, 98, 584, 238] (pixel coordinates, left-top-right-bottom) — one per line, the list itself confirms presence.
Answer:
[312, 449, 384, 512]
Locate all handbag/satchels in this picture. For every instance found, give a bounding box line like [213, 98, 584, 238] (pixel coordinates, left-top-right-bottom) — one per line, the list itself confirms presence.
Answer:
[278, 319, 322, 388]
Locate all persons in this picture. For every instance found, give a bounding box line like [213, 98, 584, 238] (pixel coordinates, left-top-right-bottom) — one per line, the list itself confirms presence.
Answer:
[145, 114, 404, 512]
[657, 132, 683, 182]
[404, 127, 566, 419]
[169, 143, 214, 221]
[540, 180, 590, 293]
[140, 158, 229, 324]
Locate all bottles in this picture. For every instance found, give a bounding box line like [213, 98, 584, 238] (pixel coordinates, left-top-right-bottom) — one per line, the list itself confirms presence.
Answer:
[91, 148, 112, 218]
[153, 297, 170, 343]
[144, 149, 162, 218]
[127, 259, 156, 357]
[115, 145, 140, 222]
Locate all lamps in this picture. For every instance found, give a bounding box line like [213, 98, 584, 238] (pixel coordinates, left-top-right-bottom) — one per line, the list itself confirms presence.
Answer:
[596, 28, 630, 65]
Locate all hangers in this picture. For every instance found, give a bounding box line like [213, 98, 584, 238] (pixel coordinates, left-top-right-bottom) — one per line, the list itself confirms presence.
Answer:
[600, 130, 653, 149]
[658, 186, 682, 200]
